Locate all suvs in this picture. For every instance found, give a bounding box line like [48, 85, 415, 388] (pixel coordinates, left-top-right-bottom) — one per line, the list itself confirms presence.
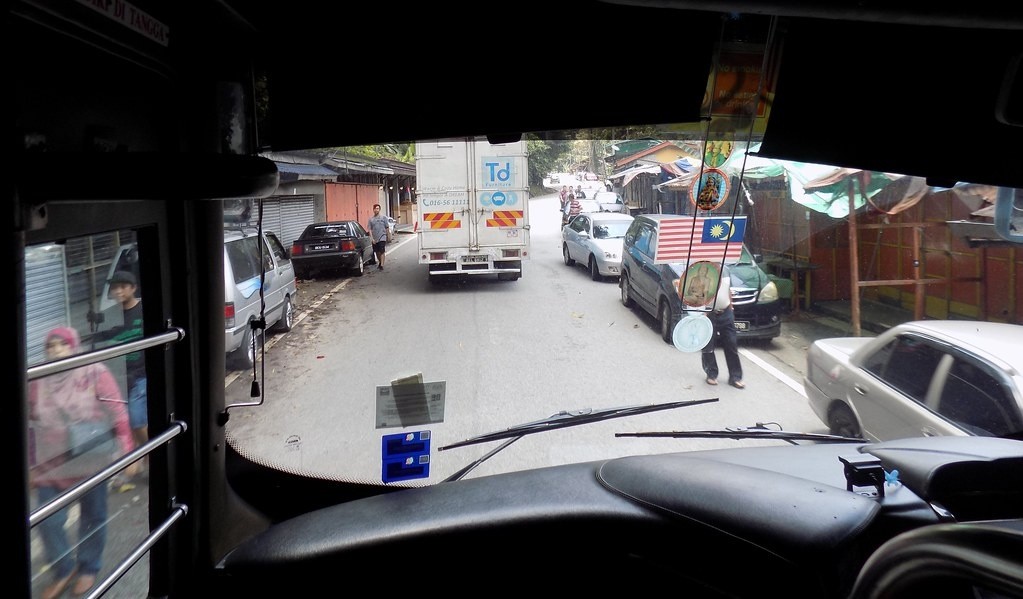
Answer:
[621, 214, 783, 347]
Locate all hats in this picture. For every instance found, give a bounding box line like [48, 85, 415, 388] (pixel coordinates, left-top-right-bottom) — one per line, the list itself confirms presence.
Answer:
[108, 271, 137, 285]
[46, 326, 81, 352]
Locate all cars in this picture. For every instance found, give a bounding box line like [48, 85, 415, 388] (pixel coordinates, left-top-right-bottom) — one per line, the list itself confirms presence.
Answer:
[584, 172, 597, 181]
[292, 220, 375, 279]
[562, 211, 636, 281]
[563, 188, 632, 226]
[801, 320, 1023, 448]
[550, 175, 560, 184]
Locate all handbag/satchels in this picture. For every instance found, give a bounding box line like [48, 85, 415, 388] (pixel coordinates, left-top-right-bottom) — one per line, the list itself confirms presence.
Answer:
[65, 425, 118, 462]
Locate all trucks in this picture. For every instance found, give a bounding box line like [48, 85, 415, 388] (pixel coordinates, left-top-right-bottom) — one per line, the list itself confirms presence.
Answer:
[415, 132, 532, 283]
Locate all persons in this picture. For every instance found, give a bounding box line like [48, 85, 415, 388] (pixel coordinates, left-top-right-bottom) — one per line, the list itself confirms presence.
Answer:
[25, 325, 133, 599]
[702, 261, 745, 388]
[558, 184, 585, 224]
[93, 273, 150, 491]
[368, 204, 390, 271]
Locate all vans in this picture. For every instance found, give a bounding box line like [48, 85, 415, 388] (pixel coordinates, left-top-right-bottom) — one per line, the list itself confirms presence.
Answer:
[224, 223, 295, 371]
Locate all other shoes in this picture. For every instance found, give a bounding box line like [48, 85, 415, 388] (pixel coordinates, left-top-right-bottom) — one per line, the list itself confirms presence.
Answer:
[729, 380, 745, 389]
[377, 262, 384, 270]
[72, 572, 97, 597]
[40, 562, 80, 599]
[705, 375, 718, 385]
